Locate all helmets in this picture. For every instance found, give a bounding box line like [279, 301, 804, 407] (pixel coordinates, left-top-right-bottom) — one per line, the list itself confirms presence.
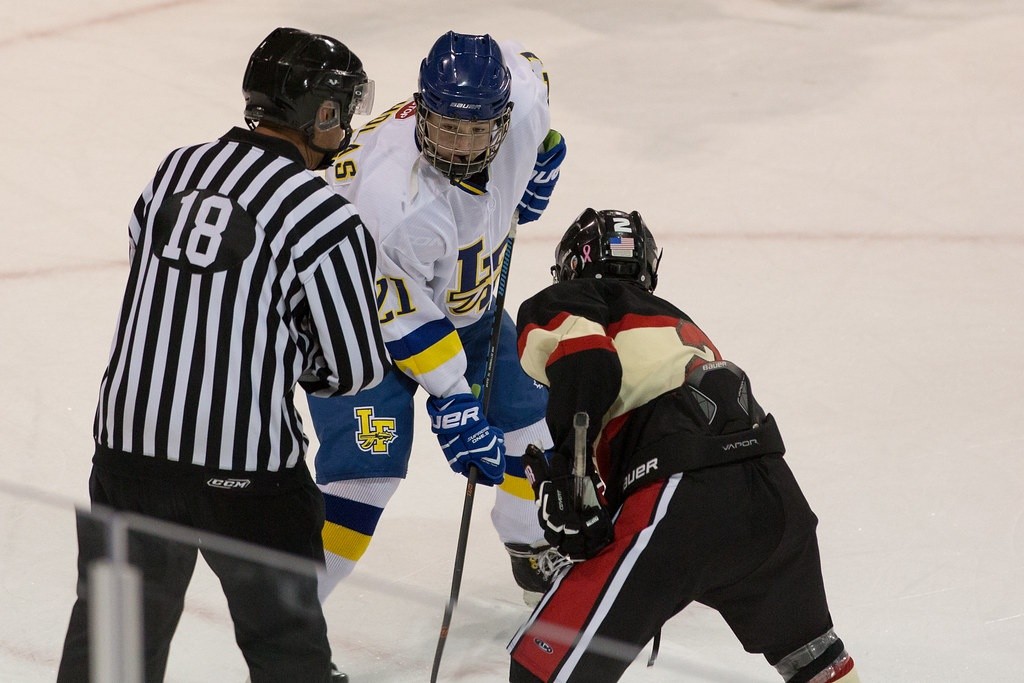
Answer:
[242, 27, 368, 153]
[412, 29, 516, 181]
[550, 209, 661, 293]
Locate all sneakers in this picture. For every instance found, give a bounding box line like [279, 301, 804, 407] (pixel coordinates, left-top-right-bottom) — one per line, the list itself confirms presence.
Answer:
[504, 542, 572, 607]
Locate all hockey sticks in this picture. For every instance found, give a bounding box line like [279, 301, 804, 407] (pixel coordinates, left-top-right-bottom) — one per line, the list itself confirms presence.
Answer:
[429, 206, 591, 683]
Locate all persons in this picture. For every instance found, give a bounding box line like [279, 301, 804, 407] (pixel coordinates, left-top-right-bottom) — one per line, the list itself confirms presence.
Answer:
[317, 31, 566, 608]
[507, 208, 861, 683]
[55, 26, 391, 683]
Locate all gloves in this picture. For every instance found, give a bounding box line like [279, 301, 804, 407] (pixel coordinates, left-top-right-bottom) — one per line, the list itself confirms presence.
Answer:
[515, 127, 570, 226]
[426, 394, 505, 487]
[522, 444, 616, 560]
